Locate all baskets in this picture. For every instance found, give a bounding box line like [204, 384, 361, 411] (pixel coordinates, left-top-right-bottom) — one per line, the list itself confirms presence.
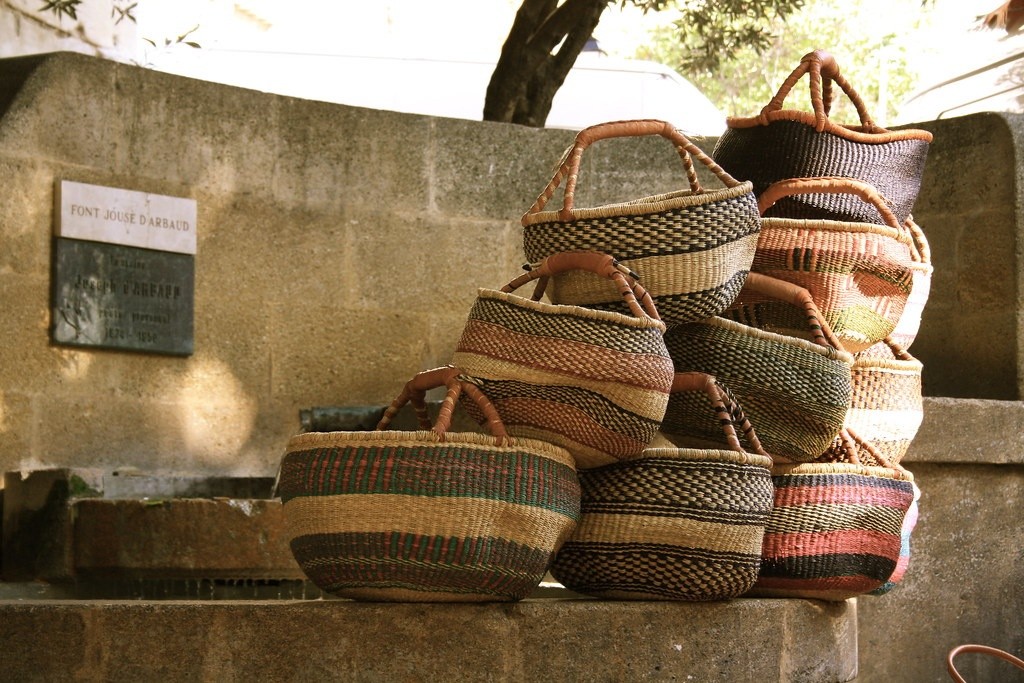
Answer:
[853, 216, 935, 360]
[449, 249, 675, 469]
[279, 365, 584, 603]
[712, 51, 934, 222]
[752, 410, 915, 601]
[547, 370, 778, 602]
[659, 269, 850, 464]
[838, 333, 928, 468]
[860, 457, 922, 596]
[522, 118, 758, 331]
[734, 174, 911, 353]
